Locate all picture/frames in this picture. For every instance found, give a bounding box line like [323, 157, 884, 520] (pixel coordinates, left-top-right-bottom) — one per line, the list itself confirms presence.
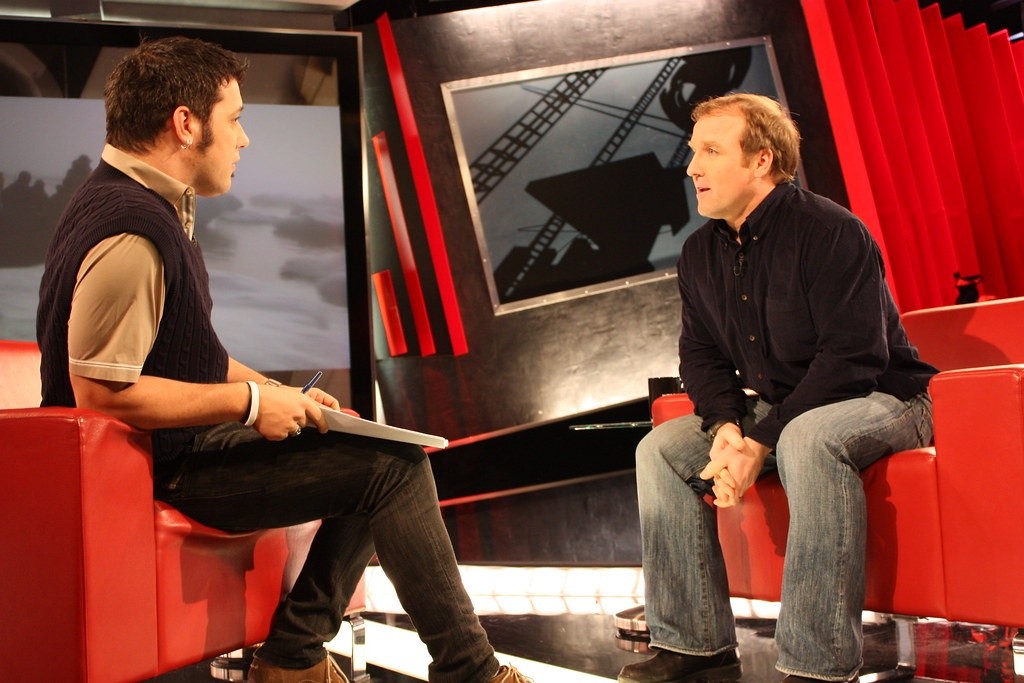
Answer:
[439, 34, 808, 316]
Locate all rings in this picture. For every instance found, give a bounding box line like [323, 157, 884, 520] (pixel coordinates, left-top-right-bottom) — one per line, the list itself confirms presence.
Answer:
[291, 425, 302, 437]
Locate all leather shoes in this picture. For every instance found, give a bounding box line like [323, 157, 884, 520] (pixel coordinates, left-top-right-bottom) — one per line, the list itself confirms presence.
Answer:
[782, 674, 860, 683]
[618, 649, 742, 683]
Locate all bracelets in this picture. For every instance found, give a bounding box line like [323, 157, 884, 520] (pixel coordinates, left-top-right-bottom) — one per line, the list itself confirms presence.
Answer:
[243, 381, 259, 426]
[706, 417, 741, 443]
[265, 379, 282, 387]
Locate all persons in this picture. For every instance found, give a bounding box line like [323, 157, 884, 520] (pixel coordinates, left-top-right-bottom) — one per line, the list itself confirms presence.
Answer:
[37, 37, 539, 683]
[618, 90, 941, 683]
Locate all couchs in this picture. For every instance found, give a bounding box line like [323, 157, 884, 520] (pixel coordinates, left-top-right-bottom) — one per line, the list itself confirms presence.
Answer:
[651, 295, 1024, 680]
[1, 406, 371, 683]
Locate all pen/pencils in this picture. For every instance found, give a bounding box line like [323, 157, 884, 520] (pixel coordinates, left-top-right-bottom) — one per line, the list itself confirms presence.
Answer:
[301, 370, 323, 395]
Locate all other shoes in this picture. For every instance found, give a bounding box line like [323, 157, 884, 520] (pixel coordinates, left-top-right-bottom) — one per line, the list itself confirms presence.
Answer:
[490, 663, 534, 683]
[247, 649, 350, 683]
